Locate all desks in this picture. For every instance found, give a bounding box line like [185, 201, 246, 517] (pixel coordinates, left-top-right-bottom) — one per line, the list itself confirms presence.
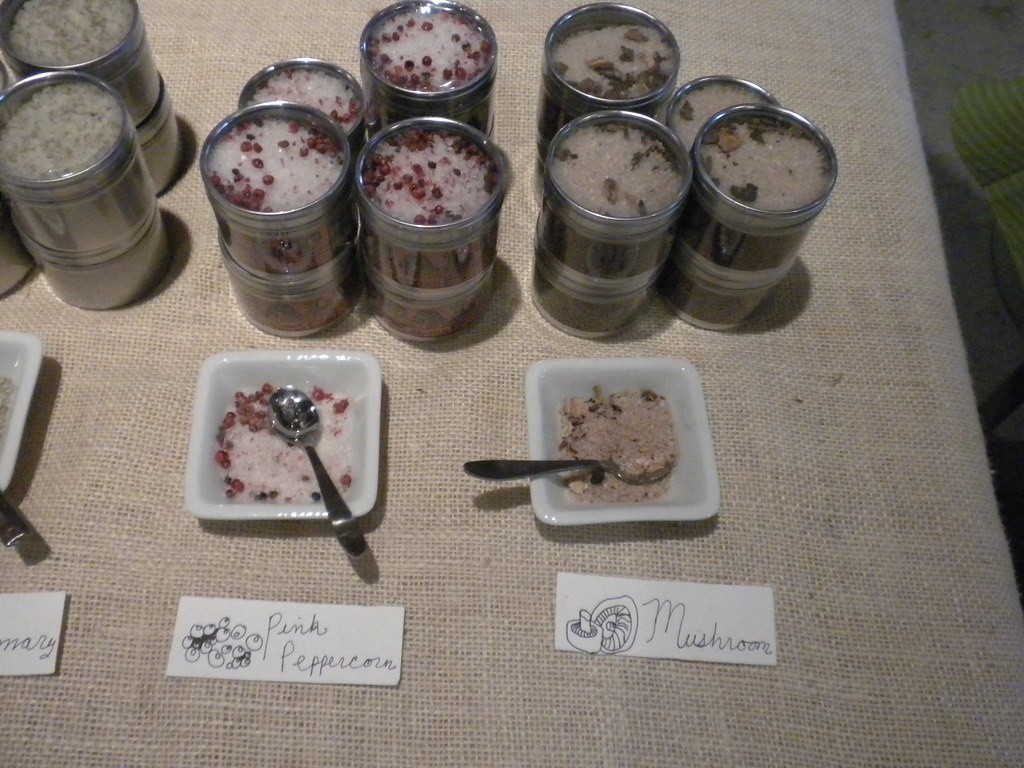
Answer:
[0, 2, 1024, 766]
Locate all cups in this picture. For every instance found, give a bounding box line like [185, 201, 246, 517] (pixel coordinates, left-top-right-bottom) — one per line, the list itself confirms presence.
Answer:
[660, 72, 785, 155]
[530, 1, 685, 180]
[1, 0, 185, 198]
[200, 100, 361, 338]
[1, 69, 173, 311]
[357, 0, 498, 145]
[656, 100, 836, 331]
[528, 106, 694, 341]
[0, 63, 36, 295]
[351, 116, 509, 344]
[237, 54, 362, 154]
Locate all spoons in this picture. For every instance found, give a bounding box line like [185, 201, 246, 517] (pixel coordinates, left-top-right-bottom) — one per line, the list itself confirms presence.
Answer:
[265, 384, 370, 562]
[459, 447, 672, 484]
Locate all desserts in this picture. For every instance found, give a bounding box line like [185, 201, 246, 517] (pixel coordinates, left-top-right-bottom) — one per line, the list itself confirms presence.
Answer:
[0, 2, 832, 510]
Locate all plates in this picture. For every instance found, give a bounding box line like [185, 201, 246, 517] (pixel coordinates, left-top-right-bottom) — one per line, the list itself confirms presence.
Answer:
[184, 350, 384, 521]
[0, 331, 47, 495]
[526, 355, 724, 527]
